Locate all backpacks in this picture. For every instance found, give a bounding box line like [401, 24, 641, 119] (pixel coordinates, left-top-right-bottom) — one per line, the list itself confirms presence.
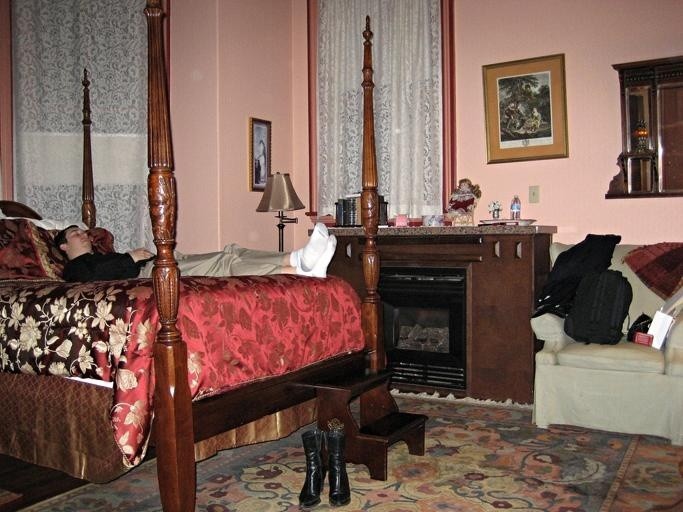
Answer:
[564, 270, 632, 344]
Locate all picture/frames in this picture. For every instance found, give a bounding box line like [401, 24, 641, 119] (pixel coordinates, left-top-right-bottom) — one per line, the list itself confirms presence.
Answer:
[247, 115, 274, 193]
[481, 52, 570, 166]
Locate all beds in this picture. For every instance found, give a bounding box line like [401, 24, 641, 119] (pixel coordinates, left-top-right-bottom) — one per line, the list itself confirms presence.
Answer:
[0, 0, 390, 512]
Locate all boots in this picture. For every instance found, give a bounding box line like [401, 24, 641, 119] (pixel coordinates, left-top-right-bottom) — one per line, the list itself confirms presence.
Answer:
[299, 429, 325, 508]
[325, 428, 351, 508]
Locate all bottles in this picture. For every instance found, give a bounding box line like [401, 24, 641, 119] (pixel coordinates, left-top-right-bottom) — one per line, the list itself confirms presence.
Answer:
[510, 194, 521, 220]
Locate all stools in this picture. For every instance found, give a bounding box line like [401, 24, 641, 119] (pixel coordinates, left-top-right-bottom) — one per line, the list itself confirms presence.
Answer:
[307, 358, 428, 483]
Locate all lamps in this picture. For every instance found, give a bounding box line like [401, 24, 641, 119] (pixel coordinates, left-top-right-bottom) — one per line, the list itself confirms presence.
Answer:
[255, 172, 306, 253]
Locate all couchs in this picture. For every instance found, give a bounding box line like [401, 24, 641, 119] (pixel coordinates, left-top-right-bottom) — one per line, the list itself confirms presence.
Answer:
[529, 239, 683, 446]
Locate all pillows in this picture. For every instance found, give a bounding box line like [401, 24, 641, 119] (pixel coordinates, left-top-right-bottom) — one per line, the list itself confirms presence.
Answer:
[32, 205, 120, 272]
[2, 196, 43, 224]
[0, 216, 49, 281]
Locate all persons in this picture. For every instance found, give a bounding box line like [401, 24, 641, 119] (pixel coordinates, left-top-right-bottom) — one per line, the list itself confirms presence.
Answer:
[447, 179, 483, 212]
[55, 222, 337, 284]
[254, 141, 267, 184]
